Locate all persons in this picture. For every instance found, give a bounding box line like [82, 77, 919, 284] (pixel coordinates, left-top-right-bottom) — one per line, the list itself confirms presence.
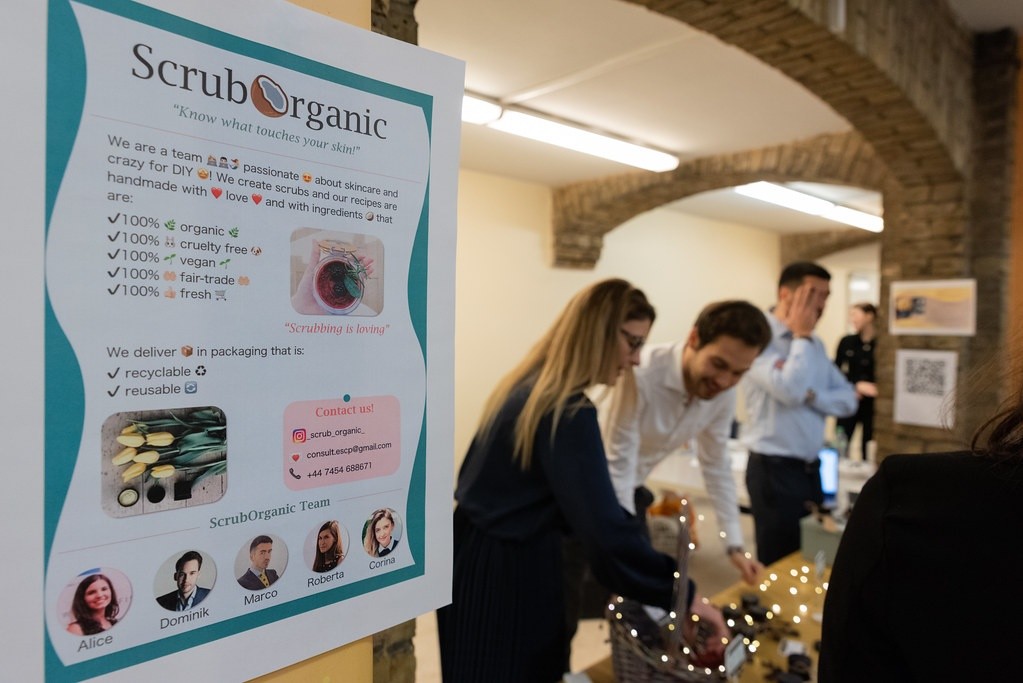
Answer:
[363, 509, 399, 557]
[819, 371, 1023, 682]
[68, 575, 117, 635]
[312, 521, 343, 574]
[831, 303, 877, 462]
[738, 261, 857, 567]
[156, 551, 211, 611]
[592, 300, 769, 612]
[437, 279, 730, 682]
[236, 536, 279, 590]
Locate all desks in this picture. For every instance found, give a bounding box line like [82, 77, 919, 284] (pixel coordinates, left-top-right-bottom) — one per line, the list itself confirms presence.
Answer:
[569, 546, 829, 683]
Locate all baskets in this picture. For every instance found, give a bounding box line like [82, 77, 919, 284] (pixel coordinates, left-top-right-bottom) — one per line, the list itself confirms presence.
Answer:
[604, 505, 726, 683]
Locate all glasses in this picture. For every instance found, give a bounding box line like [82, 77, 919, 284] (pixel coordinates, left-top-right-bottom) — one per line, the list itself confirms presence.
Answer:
[621, 329, 645, 349]
